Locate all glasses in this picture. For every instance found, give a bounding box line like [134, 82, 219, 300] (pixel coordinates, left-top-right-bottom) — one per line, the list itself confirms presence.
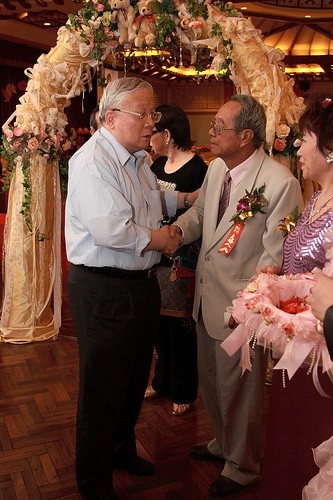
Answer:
[210, 121, 245, 135]
[111, 108, 163, 123]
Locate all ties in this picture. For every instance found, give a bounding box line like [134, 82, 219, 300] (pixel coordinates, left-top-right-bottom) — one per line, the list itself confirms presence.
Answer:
[217, 171, 234, 226]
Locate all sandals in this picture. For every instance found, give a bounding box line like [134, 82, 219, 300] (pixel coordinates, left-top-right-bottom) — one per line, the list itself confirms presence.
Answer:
[172, 403, 190, 415]
[143, 385, 157, 399]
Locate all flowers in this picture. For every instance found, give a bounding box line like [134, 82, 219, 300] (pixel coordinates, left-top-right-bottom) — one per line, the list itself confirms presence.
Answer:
[274, 124, 305, 195]
[69, 0, 243, 86]
[0, 125, 71, 240]
[274, 205, 301, 238]
[230, 181, 269, 224]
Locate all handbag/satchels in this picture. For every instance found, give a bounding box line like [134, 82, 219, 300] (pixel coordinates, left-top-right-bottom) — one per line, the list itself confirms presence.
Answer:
[155, 265, 196, 316]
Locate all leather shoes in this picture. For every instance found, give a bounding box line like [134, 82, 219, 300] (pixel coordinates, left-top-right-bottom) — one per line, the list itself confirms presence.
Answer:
[209, 474, 244, 496]
[190, 443, 216, 458]
[113, 454, 155, 475]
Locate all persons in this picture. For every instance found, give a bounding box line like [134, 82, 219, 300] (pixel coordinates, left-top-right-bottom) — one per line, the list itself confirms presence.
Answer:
[168, 94, 303, 498]
[144, 104, 209, 414]
[255, 99, 333, 500]
[88, 105, 103, 137]
[306, 273, 333, 362]
[64, 76, 201, 500]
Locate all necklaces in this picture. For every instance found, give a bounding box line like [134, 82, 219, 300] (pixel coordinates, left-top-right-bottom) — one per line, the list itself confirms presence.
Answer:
[303, 194, 333, 225]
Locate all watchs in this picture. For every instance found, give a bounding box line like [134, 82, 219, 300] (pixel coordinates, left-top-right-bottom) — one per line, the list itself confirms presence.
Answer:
[183, 193, 190, 208]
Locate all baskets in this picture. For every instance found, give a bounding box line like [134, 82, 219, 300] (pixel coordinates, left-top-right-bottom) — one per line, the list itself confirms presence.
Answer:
[244, 227, 332, 351]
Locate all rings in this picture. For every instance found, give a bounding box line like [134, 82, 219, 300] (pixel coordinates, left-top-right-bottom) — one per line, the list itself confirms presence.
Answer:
[309, 284, 314, 294]
